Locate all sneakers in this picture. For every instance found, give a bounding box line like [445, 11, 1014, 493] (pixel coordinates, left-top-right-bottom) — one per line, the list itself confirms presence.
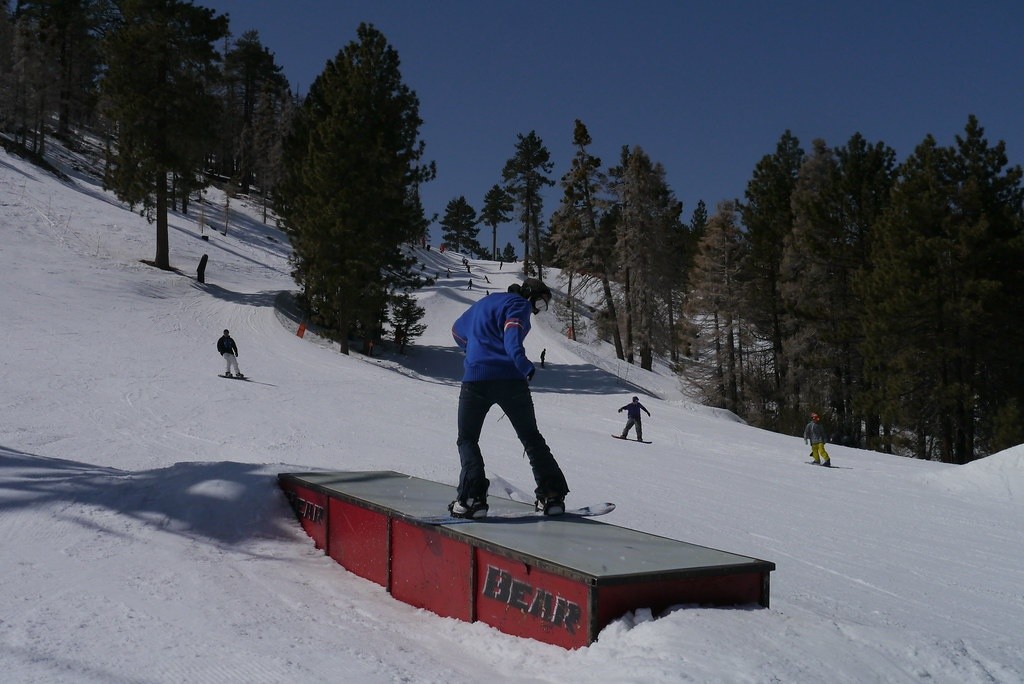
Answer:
[548, 498, 563, 515]
[452, 496, 487, 518]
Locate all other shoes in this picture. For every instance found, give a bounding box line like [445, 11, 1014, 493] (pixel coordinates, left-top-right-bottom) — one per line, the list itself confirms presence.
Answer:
[235, 373, 243, 377]
[619, 435, 626, 438]
[822, 462, 830, 465]
[812, 461, 820, 465]
[638, 439, 642, 442]
[225, 372, 233, 376]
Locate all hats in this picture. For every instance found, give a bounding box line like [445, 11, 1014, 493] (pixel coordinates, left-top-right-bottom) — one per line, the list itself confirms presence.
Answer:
[812, 413, 819, 418]
[224, 329, 229, 334]
[633, 396, 638, 402]
[522, 278, 552, 315]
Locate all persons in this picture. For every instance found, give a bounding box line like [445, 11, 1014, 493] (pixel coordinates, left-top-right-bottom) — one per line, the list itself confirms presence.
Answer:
[618, 396, 650, 441]
[217, 329, 244, 377]
[540, 349, 546, 367]
[440, 243, 444, 252]
[468, 279, 472, 289]
[445, 268, 450, 278]
[803, 413, 830, 465]
[487, 290, 489, 295]
[448, 278, 570, 520]
[422, 238, 425, 248]
[462, 258, 470, 272]
[499, 261, 503, 270]
[484, 276, 489, 282]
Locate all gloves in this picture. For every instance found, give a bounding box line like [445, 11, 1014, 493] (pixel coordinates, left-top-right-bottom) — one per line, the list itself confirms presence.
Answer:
[618, 408, 622, 413]
[235, 353, 238, 357]
[648, 413, 650, 417]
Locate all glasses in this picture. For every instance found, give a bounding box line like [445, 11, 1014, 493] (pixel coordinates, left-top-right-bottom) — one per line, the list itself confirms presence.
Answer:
[535, 298, 548, 311]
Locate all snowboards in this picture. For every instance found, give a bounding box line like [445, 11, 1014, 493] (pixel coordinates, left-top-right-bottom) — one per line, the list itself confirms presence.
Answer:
[218, 373, 251, 380]
[403, 502, 616, 526]
[805, 461, 840, 469]
[611, 434, 652, 444]
